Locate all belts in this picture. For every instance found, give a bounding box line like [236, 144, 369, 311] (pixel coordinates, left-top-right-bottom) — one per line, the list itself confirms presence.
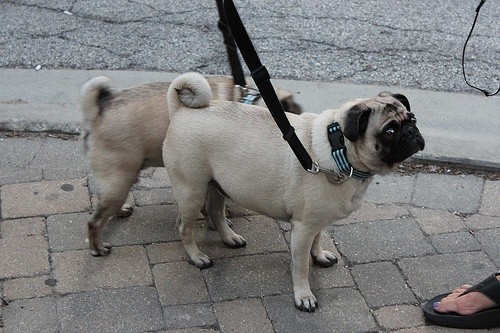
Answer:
[216, 1, 325, 173]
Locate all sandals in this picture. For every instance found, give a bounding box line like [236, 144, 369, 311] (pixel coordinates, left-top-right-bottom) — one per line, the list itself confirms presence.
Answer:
[423, 271, 500, 329]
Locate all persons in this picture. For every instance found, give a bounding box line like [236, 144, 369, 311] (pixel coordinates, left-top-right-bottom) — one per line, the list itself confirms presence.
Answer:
[423, 267, 500, 333]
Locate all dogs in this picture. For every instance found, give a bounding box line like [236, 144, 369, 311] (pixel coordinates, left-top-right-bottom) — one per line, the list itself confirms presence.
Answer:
[79, 74, 302, 257]
[161, 71, 426, 314]
